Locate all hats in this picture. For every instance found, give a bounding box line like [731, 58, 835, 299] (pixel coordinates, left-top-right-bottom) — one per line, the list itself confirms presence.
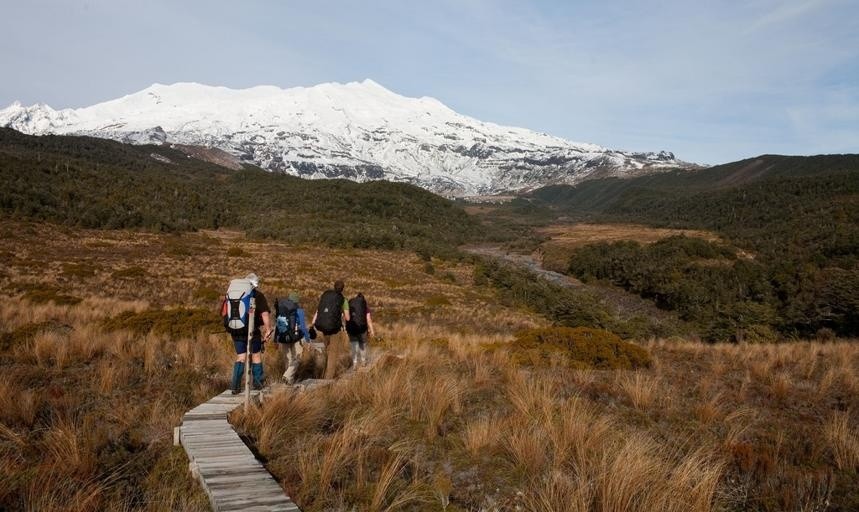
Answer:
[246, 272, 259, 287]
[288, 291, 299, 303]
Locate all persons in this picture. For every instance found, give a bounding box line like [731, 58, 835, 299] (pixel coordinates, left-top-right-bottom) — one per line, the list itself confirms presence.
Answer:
[220, 273, 272, 394]
[308, 280, 350, 380]
[344, 285, 374, 372]
[272, 288, 312, 387]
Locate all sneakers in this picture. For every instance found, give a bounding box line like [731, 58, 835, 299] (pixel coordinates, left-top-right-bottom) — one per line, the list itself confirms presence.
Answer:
[232, 388, 240, 394]
[255, 384, 265, 390]
[282, 375, 293, 386]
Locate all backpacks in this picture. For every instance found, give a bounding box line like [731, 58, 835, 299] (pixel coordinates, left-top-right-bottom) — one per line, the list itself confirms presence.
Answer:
[346, 297, 367, 334]
[315, 290, 343, 335]
[224, 278, 255, 333]
[276, 300, 297, 342]
